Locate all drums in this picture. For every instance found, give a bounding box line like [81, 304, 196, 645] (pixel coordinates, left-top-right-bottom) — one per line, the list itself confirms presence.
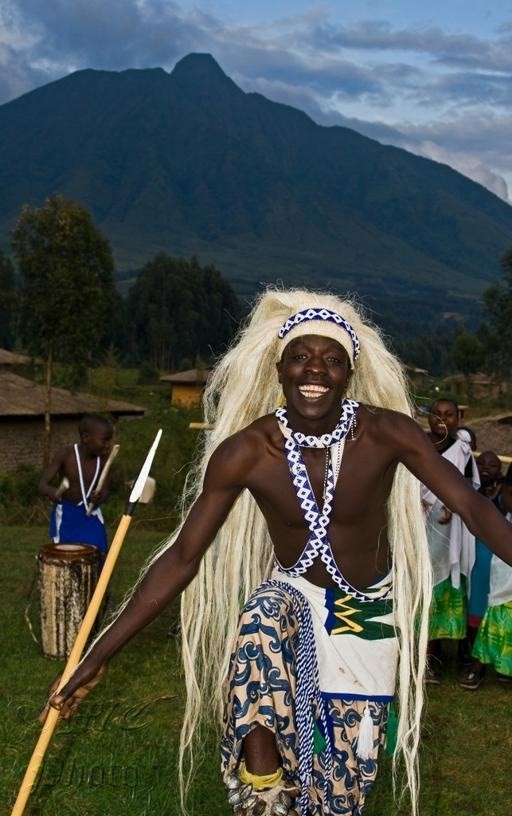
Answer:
[38, 542, 101, 659]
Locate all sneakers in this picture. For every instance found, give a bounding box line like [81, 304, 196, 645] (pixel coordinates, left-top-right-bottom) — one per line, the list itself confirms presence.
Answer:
[424, 662, 442, 687]
[457, 658, 487, 691]
[496, 675, 512, 684]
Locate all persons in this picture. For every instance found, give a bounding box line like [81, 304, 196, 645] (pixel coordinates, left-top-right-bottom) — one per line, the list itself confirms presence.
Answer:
[470, 450, 502, 486]
[55, 289, 512, 816]
[416, 398, 479, 680]
[40, 415, 114, 562]
[460, 461, 512, 690]
